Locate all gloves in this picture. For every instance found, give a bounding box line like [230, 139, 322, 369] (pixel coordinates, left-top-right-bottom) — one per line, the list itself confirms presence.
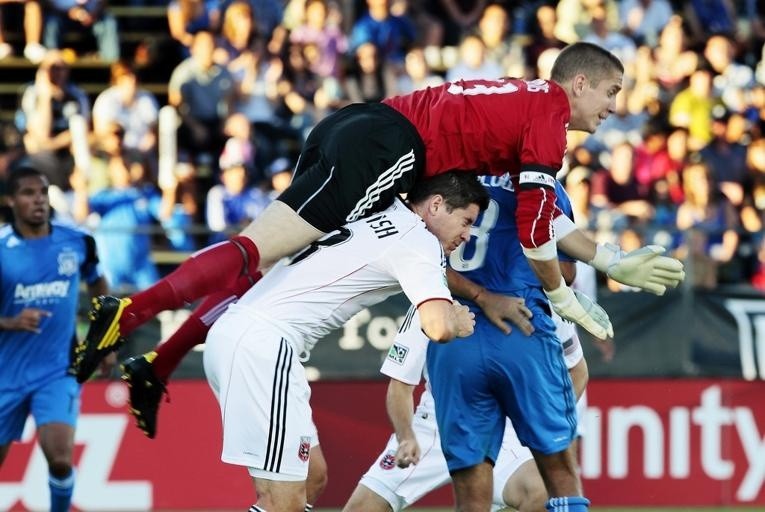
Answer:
[544, 274, 616, 342]
[589, 240, 687, 299]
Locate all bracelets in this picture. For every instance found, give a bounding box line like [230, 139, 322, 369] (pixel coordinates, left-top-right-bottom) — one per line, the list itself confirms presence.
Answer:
[473, 288, 484, 300]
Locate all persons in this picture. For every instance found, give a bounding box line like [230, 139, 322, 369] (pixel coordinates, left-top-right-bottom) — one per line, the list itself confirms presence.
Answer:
[202, 166, 536, 512]
[0, 164, 116, 512]
[0, 0, 765, 294]
[74, 41, 686, 439]
[341, 300, 589, 512]
[424, 169, 590, 512]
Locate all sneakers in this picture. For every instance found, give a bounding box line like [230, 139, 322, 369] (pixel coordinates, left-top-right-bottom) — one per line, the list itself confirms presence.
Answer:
[117, 349, 170, 442]
[70, 293, 130, 386]
